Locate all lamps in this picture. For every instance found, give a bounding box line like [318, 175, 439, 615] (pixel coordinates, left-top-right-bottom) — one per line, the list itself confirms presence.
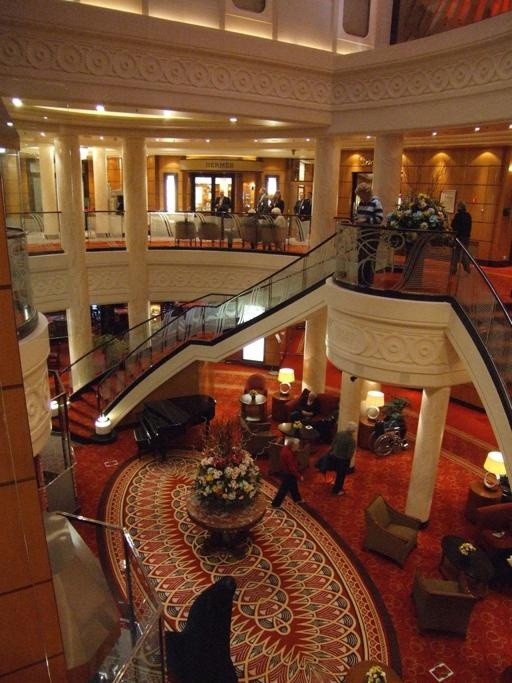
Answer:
[277, 367, 296, 394]
[365, 390, 385, 419]
[483, 451, 506, 490]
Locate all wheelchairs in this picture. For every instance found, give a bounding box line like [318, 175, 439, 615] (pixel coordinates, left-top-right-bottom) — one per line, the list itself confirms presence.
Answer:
[371, 419, 410, 455]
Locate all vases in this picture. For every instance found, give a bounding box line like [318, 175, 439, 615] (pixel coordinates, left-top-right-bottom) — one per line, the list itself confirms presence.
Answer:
[252, 395, 255, 400]
[400, 238, 427, 289]
[293, 420, 303, 430]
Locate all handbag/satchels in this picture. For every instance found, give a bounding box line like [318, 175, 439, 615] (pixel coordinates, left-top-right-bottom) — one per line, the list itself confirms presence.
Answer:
[313, 450, 336, 472]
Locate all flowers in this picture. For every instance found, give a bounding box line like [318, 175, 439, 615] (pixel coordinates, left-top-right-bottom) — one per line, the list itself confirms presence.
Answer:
[248, 389, 258, 395]
[387, 191, 451, 245]
[191, 417, 260, 510]
[367, 665, 388, 682]
[458, 541, 475, 556]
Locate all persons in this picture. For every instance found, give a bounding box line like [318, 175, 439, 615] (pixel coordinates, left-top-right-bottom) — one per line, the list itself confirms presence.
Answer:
[303, 192, 312, 214]
[255, 188, 269, 213]
[351, 181, 385, 289]
[294, 191, 306, 214]
[214, 189, 232, 214]
[326, 422, 358, 497]
[270, 436, 310, 507]
[270, 189, 285, 213]
[289, 391, 316, 425]
[448, 201, 473, 278]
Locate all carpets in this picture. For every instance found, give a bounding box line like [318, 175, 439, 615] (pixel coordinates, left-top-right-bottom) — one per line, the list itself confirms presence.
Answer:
[96, 446, 403, 682]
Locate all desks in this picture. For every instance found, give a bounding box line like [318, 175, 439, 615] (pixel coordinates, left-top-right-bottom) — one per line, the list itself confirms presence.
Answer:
[272, 391, 294, 419]
[186, 493, 267, 562]
[441, 535, 495, 598]
[239, 393, 268, 420]
[465, 481, 503, 511]
[343, 655, 401, 682]
[220, 226, 241, 249]
[278, 423, 319, 451]
[359, 415, 385, 450]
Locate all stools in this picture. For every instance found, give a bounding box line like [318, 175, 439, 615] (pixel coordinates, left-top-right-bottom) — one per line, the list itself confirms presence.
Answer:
[133, 429, 154, 459]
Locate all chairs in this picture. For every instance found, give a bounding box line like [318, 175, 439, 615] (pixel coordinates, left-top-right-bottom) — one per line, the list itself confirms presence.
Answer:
[475, 502, 512, 558]
[174, 221, 197, 246]
[411, 566, 474, 638]
[285, 391, 344, 441]
[363, 494, 422, 564]
[261, 441, 314, 476]
[260, 224, 282, 250]
[242, 373, 268, 416]
[238, 222, 261, 247]
[198, 222, 222, 248]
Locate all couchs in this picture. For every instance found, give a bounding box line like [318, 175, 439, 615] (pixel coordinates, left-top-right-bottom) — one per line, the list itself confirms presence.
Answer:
[237, 417, 277, 451]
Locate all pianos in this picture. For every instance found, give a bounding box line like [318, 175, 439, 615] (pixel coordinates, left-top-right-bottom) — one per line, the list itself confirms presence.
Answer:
[133, 394, 215, 458]
